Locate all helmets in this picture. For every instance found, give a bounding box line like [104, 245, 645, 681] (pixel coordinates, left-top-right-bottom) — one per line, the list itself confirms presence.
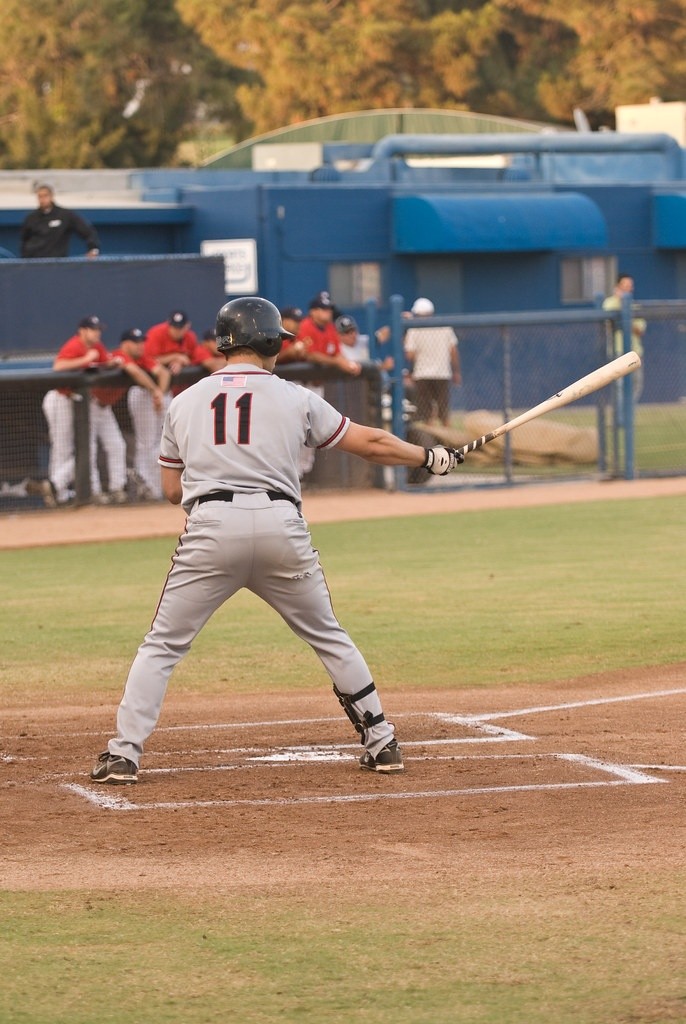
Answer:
[215, 296, 294, 357]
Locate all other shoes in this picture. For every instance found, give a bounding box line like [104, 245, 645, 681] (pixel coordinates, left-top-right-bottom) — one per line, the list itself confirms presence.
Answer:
[43, 479, 60, 508]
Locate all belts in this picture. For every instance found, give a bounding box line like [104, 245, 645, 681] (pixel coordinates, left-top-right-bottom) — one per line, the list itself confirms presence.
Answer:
[199, 491, 295, 505]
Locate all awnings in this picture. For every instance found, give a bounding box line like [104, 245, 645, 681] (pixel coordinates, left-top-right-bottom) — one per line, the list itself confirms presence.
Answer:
[391, 193, 606, 254]
[650, 193, 686, 249]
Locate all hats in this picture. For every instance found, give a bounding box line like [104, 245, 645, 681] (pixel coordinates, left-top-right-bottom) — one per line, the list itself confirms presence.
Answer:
[120, 329, 147, 343]
[80, 315, 105, 329]
[170, 311, 189, 327]
[310, 294, 336, 308]
[411, 298, 435, 314]
[337, 316, 356, 331]
[283, 309, 304, 321]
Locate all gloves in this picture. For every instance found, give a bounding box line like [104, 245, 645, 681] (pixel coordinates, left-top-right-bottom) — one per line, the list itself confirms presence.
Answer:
[421, 444, 465, 476]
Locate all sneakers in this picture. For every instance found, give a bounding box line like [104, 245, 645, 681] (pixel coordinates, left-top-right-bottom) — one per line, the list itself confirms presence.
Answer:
[359, 741, 404, 771]
[90, 751, 139, 784]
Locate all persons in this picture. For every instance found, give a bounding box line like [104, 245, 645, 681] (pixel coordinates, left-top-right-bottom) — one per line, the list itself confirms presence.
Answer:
[91, 296, 467, 784]
[20, 187, 101, 256]
[40, 291, 462, 510]
[601, 276, 647, 424]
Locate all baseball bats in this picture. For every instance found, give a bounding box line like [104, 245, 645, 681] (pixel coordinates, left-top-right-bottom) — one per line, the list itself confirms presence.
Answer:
[456, 350, 643, 456]
